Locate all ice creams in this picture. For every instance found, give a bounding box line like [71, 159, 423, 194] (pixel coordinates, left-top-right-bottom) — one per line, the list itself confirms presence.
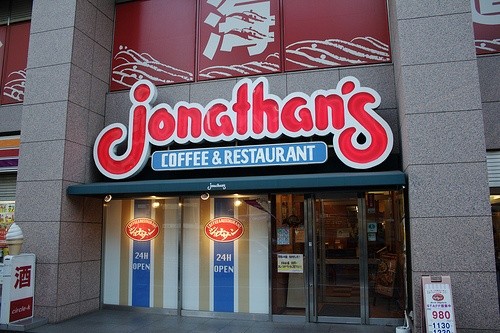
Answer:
[6, 223, 23, 256]
